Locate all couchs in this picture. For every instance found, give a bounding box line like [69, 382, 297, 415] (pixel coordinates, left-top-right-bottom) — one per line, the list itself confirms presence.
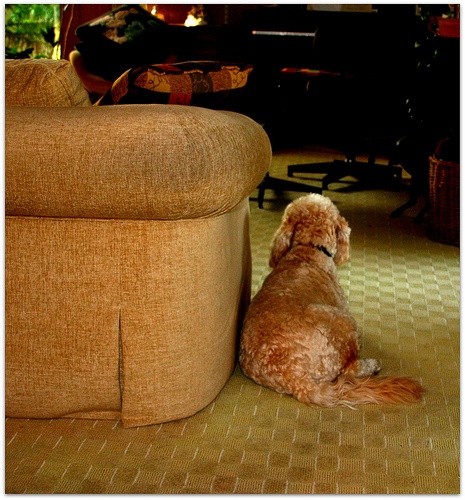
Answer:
[5, 58, 271, 429]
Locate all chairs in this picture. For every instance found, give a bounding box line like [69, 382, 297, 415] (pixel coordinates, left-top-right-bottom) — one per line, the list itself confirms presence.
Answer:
[279, 59, 404, 191]
[248, 171, 322, 211]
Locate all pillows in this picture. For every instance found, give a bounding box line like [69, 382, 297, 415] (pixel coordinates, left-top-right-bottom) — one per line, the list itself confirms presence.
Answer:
[5, 58, 92, 107]
[76, 42, 124, 82]
[94, 61, 259, 115]
[75, 4, 181, 66]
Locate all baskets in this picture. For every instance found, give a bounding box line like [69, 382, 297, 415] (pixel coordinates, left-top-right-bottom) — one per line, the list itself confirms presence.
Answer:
[427, 135, 459, 241]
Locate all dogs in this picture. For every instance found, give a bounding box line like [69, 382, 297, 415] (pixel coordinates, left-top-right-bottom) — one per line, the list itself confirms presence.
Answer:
[236, 192, 426, 409]
